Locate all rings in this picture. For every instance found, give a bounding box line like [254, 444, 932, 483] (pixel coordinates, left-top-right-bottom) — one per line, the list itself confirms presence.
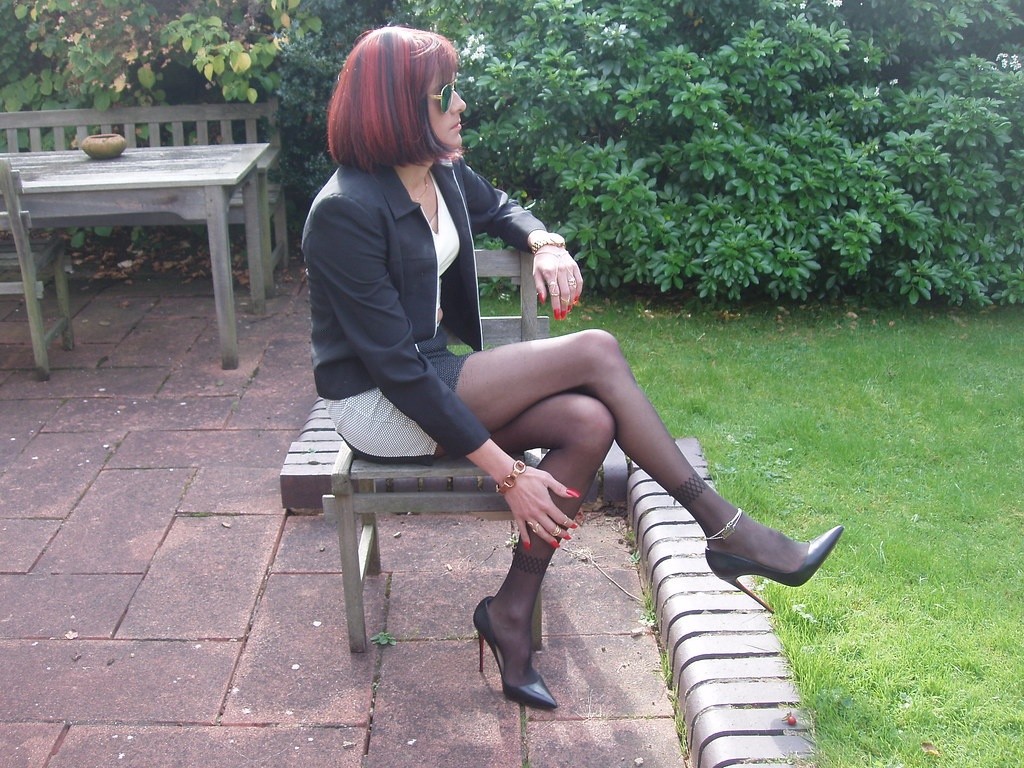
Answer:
[563, 514, 569, 527]
[568, 277, 577, 289]
[550, 525, 562, 537]
[561, 295, 571, 304]
[527, 521, 540, 533]
[548, 282, 560, 296]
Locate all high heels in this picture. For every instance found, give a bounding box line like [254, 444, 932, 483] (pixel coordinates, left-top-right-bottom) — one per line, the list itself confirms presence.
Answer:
[473, 597, 557, 709]
[703, 524, 844, 610]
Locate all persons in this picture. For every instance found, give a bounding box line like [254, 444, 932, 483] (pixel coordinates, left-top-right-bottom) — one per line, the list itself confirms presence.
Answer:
[301, 27, 846, 710]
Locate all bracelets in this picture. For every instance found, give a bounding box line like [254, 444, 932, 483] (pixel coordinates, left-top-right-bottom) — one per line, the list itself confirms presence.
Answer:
[496, 460, 526, 496]
[534, 249, 569, 259]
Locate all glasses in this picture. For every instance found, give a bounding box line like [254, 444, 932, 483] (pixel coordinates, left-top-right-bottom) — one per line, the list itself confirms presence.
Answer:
[428, 79, 458, 114]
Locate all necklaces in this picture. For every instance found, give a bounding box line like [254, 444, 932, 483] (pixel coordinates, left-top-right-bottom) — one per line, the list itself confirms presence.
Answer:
[414, 175, 429, 202]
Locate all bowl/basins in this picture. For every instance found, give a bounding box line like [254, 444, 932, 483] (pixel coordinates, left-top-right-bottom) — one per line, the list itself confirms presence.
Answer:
[81, 134, 127, 159]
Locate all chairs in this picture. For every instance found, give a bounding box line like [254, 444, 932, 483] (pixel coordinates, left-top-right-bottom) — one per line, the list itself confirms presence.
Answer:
[320, 242, 555, 661]
[3, 153, 79, 386]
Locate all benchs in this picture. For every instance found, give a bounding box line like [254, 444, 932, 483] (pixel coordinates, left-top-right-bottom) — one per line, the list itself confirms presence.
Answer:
[1, 103, 292, 302]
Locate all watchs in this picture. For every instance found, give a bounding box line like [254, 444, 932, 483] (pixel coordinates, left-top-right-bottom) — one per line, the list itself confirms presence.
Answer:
[531, 232, 566, 253]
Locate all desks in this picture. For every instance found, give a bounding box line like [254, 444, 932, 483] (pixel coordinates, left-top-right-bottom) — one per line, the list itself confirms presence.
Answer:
[2, 147, 278, 369]
